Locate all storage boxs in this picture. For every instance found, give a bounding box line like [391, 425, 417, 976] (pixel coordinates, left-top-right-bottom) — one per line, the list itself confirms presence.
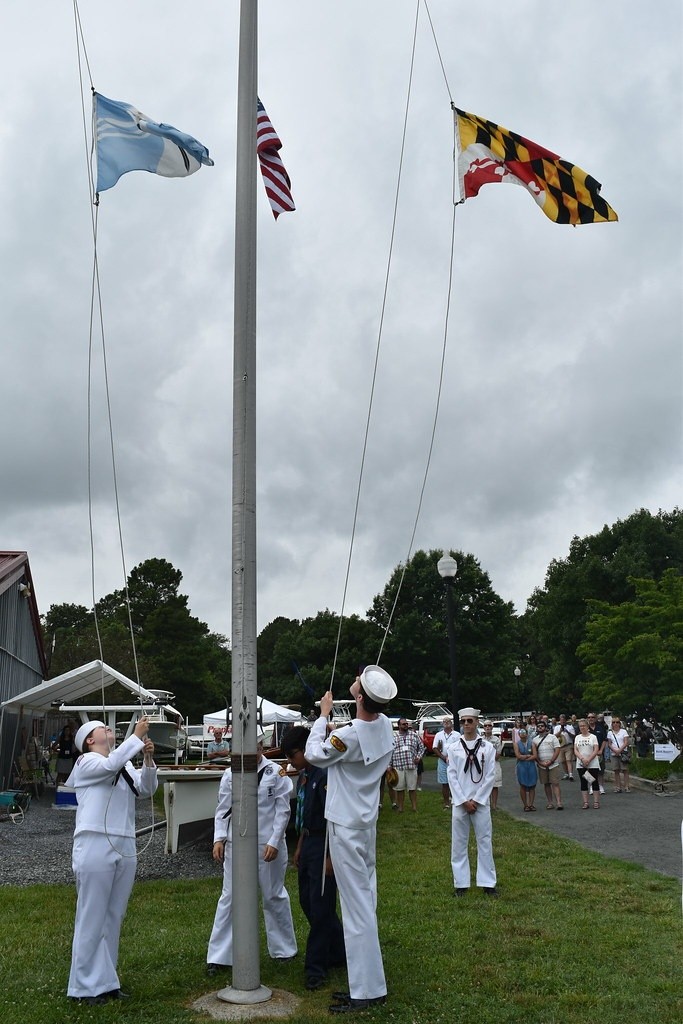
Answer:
[55, 784, 77, 807]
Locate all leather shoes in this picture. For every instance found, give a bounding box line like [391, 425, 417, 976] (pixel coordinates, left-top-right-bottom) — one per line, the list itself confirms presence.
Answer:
[68, 960, 387, 1013]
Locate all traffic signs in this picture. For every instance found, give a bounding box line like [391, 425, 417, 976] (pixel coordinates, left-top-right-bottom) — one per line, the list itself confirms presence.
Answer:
[654, 741, 680, 762]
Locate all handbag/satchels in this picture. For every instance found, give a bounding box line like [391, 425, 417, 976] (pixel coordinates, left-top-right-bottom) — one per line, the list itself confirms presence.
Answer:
[621, 751, 631, 765]
[417, 759, 424, 773]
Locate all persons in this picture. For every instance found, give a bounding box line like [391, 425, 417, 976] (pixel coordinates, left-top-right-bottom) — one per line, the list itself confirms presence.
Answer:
[306, 710, 317, 722]
[432, 717, 462, 811]
[446, 708, 496, 897]
[52, 725, 77, 788]
[378, 719, 426, 813]
[206, 724, 299, 978]
[480, 713, 665, 811]
[208, 729, 229, 770]
[64, 720, 158, 1004]
[304, 665, 398, 1013]
[281, 726, 346, 990]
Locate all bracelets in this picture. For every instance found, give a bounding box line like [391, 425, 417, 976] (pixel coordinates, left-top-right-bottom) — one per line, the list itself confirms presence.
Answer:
[581, 758, 584, 761]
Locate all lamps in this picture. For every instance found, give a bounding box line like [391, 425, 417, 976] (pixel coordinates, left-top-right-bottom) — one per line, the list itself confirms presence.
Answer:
[19, 581, 32, 600]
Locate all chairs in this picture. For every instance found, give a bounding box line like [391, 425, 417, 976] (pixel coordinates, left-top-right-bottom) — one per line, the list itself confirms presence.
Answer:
[13, 760, 44, 801]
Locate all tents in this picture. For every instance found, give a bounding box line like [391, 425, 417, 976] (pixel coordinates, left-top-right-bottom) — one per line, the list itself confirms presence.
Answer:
[202, 695, 301, 763]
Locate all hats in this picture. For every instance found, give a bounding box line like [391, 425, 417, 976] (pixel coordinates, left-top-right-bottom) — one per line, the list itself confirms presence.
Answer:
[557, 735, 565, 748]
[360, 665, 398, 703]
[387, 768, 400, 789]
[74, 720, 104, 753]
[457, 707, 481, 721]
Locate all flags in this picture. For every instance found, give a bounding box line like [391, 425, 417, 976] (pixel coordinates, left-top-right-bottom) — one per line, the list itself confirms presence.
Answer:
[454, 107, 620, 227]
[91, 92, 214, 206]
[257, 97, 296, 220]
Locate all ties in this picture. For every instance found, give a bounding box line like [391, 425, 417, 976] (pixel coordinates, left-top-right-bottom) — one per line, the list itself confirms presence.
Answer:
[222, 767, 265, 820]
[112, 767, 141, 797]
[460, 737, 483, 775]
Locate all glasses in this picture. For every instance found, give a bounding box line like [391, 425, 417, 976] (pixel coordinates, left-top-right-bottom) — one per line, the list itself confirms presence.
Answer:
[459, 718, 476, 724]
[284, 748, 302, 761]
[612, 721, 620, 724]
[484, 725, 492, 727]
[215, 732, 222, 735]
[587, 716, 595, 719]
[519, 729, 527, 733]
[537, 725, 545, 728]
[257, 746, 263, 754]
[401, 723, 408, 726]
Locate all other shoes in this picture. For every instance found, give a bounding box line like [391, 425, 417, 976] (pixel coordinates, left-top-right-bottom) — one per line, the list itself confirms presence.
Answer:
[450, 888, 467, 898]
[377, 787, 452, 812]
[483, 887, 498, 897]
[489, 773, 632, 812]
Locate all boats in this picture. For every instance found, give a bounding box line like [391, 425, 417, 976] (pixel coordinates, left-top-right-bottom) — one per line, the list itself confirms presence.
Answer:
[116, 686, 537, 755]
